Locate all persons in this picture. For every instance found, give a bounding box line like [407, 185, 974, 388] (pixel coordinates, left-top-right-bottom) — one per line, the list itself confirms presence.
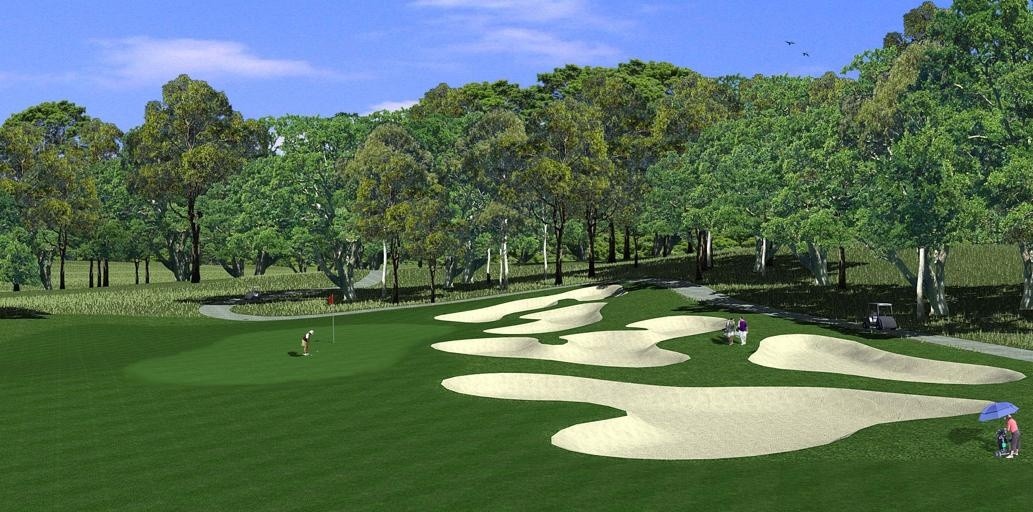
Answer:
[301, 329, 315, 356]
[725, 317, 736, 345]
[738, 316, 749, 345]
[1005, 414, 1020, 459]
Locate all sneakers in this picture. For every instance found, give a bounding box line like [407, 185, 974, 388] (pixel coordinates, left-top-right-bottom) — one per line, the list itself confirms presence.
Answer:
[1006, 452, 1020, 459]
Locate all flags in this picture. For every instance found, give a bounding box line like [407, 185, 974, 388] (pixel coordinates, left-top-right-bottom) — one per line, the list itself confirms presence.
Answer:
[327, 293, 333, 305]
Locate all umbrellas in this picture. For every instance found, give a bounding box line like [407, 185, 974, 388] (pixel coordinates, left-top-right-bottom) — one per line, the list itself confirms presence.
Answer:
[978, 402, 1019, 421]
